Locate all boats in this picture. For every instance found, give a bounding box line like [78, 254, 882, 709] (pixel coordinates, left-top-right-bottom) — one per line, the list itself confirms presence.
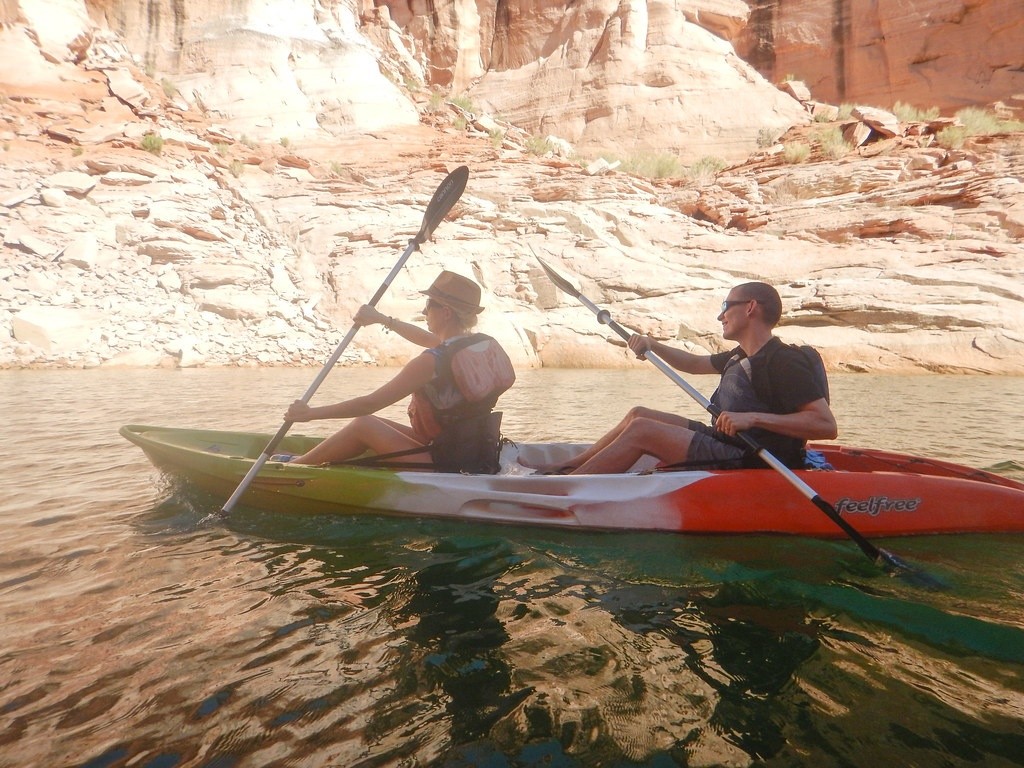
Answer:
[119, 424, 1024, 539]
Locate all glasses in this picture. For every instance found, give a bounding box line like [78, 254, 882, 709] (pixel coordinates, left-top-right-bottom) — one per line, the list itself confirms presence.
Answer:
[721, 300, 766, 314]
[425, 299, 442, 311]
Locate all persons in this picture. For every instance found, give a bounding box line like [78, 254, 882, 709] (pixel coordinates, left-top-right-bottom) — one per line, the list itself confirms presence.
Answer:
[270, 270, 516, 473]
[517, 282, 837, 475]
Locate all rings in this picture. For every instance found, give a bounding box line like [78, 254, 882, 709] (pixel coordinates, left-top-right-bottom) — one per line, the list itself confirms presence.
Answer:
[724, 427, 729, 431]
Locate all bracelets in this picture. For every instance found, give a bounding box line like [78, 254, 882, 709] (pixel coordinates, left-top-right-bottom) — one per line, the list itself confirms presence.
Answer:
[382, 316, 399, 334]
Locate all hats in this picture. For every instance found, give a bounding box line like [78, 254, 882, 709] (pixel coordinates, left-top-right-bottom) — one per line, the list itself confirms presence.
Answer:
[418, 270, 485, 314]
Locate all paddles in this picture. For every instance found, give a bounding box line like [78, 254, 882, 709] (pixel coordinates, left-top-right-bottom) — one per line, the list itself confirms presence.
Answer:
[196, 159, 479, 532]
[515, 237, 958, 599]
